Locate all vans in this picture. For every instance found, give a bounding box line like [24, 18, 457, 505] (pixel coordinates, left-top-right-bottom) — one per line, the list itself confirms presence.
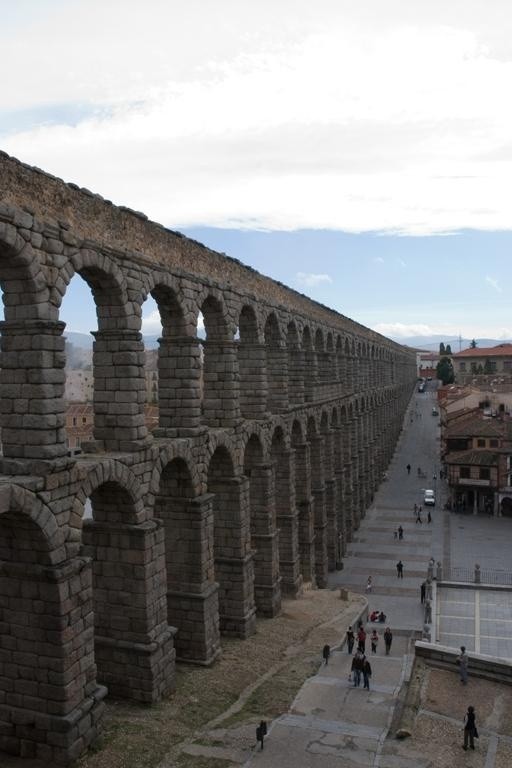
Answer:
[424, 489, 435, 505]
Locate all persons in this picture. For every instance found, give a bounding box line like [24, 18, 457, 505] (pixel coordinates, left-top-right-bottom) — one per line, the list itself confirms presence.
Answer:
[457, 646, 469, 685]
[393, 504, 433, 541]
[406, 464, 411, 473]
[461, 706, 476, 751]
[396, 560, 403, 578]
[364, 575, 373, 594]
[420, 583, 425, 604]
[342, 611, 393, 692]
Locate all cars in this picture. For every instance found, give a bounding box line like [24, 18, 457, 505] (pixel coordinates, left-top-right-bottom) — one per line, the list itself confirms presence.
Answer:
[416, 374, 433, 392]
